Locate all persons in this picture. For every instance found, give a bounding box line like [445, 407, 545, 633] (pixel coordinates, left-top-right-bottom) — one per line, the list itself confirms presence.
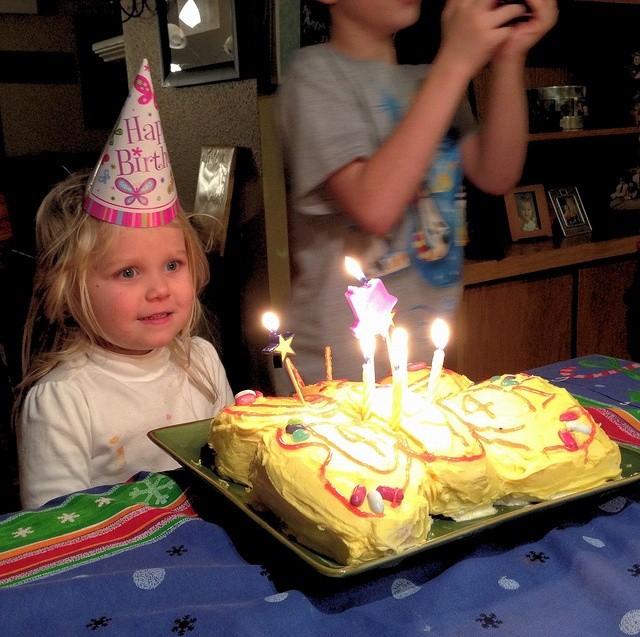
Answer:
[624, 180, 639, 201]
[275, 0, 558, 397]
[611, 179, 628, 200]
[561, 204, 582, 227]
[516, 194, 540, 231]
[18, 171, 235, 508]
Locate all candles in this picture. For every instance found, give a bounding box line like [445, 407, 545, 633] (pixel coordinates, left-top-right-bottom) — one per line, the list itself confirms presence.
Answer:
[358, 327, 377, 409]
[343, 253, 413, 385]
[259, 308, 302, 388]
[391, 357, 405, 425]
[425, 317, 452, 396]
[394, 326, 410, 388]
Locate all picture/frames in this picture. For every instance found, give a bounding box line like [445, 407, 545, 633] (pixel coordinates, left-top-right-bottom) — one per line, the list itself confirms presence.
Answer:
[505, 183, 553, 241]
[546, 185, 592, 237]
[154, 0, 256, 88]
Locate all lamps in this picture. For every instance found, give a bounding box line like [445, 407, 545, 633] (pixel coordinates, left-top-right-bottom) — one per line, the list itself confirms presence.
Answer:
[167, 0, 233, 64]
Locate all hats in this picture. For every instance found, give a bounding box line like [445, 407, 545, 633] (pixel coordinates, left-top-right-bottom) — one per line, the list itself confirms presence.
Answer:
[82, 59, 181, 228]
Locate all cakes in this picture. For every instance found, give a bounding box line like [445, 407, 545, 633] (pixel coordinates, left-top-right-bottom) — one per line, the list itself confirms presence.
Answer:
[206, 359, 623, 565]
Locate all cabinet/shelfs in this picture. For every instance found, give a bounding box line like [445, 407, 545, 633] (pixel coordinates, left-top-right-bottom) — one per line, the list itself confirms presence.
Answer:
[301, 0, 640, 383]
